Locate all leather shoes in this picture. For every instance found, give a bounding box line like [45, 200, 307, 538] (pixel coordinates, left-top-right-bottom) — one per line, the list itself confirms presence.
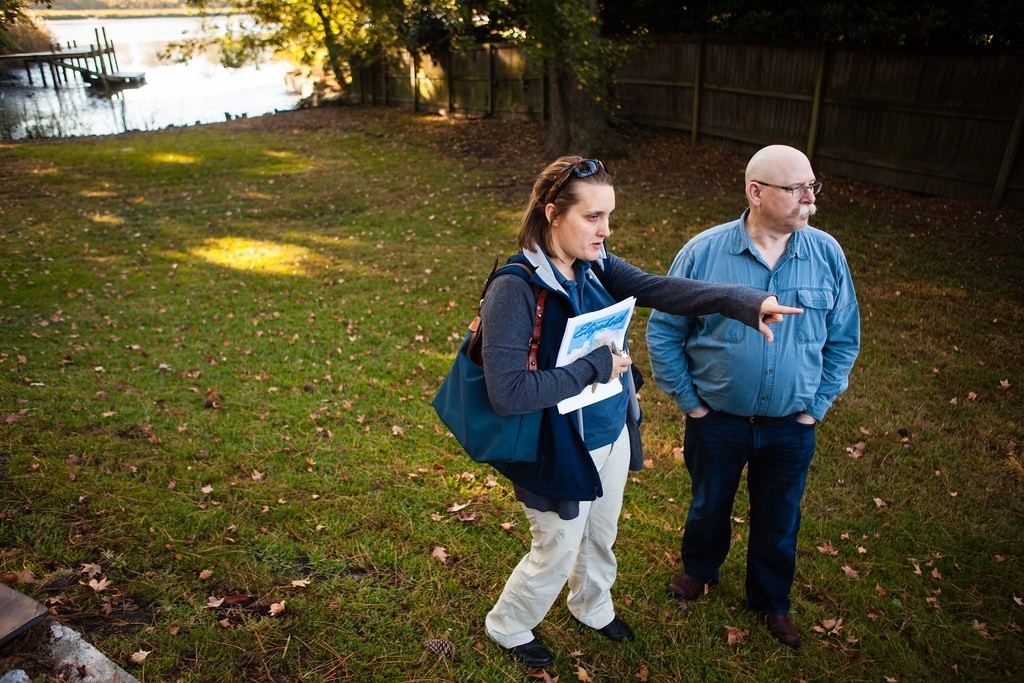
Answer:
[668, 573, 719, 602]
[755, 611, 800, 650]
[597, 615, 635, 644]
[509, 638, 554, 668]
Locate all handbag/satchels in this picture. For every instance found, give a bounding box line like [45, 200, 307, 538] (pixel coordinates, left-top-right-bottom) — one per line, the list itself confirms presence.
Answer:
[431, 257, 547, 464]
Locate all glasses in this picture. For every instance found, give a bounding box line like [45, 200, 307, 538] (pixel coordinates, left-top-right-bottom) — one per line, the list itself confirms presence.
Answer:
[750, 180, 823, 199]
[539, 158, 605, 205]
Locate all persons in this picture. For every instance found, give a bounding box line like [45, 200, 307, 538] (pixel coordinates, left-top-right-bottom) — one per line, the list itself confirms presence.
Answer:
[647, 144, 861, 647]
[486, 155, 804, 667]
